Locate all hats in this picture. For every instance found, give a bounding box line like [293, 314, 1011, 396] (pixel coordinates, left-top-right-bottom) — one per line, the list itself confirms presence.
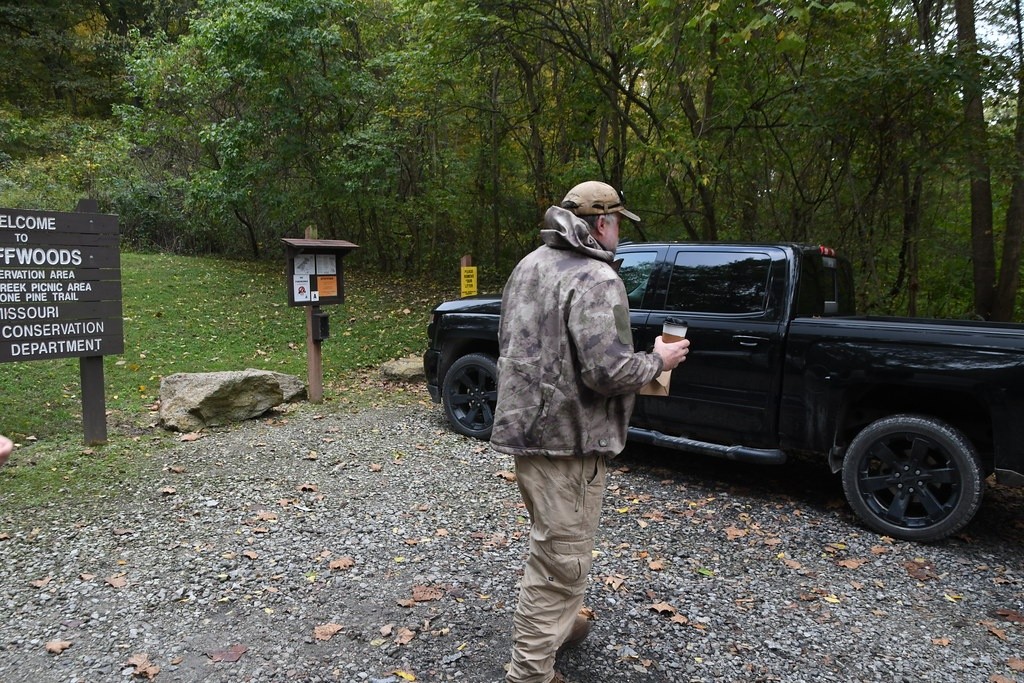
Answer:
[560, 180, 641, 222]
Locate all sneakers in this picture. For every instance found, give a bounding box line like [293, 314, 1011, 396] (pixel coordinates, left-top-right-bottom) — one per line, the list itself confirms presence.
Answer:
[555, 613, 592, 658]
[550, 673, 569, 683]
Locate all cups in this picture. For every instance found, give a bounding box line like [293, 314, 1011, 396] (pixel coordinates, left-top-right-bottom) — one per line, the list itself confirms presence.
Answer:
[662, 316, 687, 344]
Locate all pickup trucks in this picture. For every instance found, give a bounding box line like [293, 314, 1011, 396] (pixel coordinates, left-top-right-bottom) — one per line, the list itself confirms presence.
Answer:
[423, 242, 1024, 543]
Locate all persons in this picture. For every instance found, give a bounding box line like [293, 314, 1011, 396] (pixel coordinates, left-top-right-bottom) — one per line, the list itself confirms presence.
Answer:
[488, 180, 690, 683]
